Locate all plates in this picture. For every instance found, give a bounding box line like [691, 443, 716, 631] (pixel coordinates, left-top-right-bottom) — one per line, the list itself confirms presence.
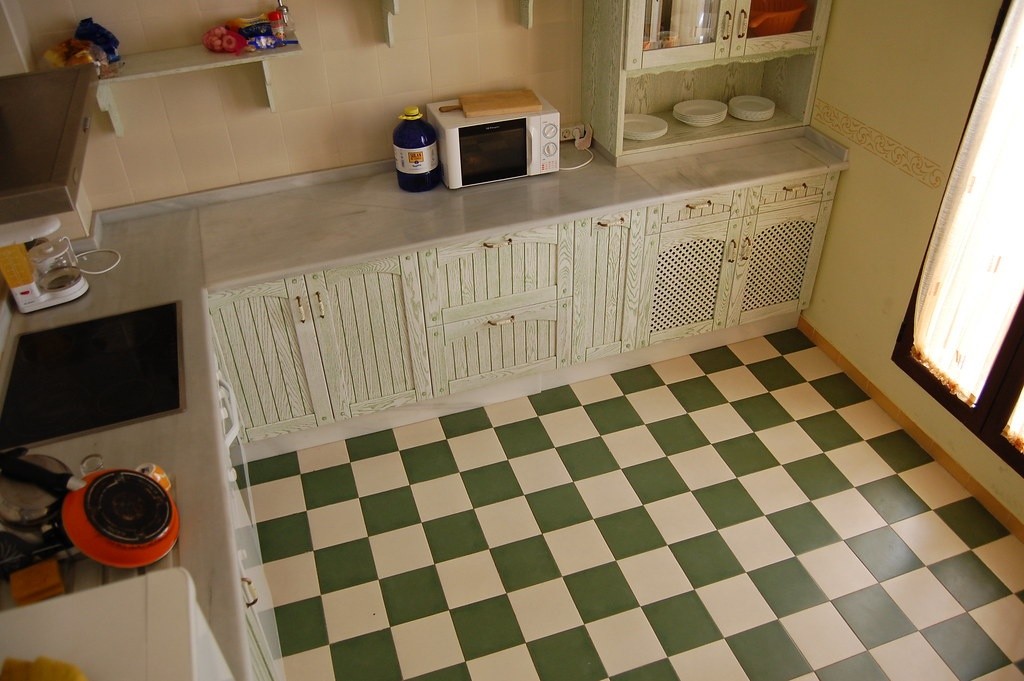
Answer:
[673, 99, 728, 127]
[623, 113, 668, 140]
[729, 96, 775, 121]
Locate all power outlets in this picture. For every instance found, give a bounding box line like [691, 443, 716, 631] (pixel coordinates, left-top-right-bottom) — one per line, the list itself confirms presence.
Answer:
[560, 122, 584, 142]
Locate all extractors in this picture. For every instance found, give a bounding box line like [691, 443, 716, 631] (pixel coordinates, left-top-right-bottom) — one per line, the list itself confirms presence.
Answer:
[0, 63, 99, 225]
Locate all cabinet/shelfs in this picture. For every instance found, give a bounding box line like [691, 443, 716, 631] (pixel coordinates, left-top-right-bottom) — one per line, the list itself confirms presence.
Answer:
[418, 210, 646, 420]
[208, 253, 417, 440]
[585, 0, 831, 166]
[229, 464, 288, 681]
[647, 175, 841, 365]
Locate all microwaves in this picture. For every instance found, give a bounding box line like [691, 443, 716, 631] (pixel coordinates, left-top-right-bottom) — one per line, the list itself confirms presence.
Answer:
[426, 89, 560, 190]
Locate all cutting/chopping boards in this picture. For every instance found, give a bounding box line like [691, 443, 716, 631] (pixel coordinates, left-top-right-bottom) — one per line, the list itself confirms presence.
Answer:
[439, 89, 543, 117]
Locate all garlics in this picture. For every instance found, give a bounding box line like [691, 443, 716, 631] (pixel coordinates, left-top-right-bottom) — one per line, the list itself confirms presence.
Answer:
[203, 26, 236, 52]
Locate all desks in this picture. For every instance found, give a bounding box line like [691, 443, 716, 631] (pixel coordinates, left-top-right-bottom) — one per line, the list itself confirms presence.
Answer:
[0, 567, 245, 681]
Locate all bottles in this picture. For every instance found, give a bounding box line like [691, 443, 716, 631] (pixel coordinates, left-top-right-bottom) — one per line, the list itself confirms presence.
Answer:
[276, 0, 288, 25]
[393, 105, 442, 192]
[267, 11, 287, 47]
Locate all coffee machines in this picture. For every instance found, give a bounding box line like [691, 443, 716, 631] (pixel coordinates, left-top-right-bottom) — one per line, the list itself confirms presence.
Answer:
[0, 216, 89, 313]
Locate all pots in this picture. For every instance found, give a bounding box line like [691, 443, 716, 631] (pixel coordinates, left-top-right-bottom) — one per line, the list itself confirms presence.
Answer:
[0, 452, 180, 568]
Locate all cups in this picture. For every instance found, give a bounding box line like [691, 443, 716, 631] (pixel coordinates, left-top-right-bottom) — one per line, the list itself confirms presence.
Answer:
[80, 454, 105, 476]
[136, 463, 171, 491]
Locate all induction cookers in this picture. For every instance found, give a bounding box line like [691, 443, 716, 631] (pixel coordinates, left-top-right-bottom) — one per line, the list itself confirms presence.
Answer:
[0, 299, 187, 452]
